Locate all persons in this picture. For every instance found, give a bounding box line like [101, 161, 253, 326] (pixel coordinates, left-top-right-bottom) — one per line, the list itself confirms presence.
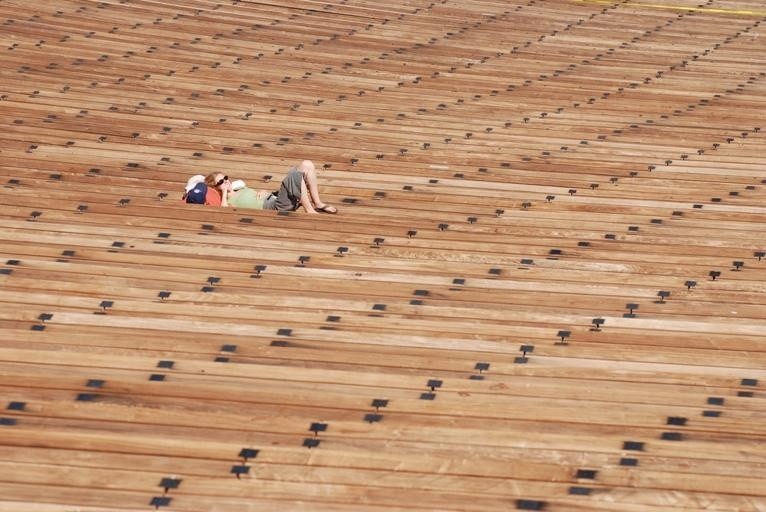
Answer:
[206, 160, 335, 215]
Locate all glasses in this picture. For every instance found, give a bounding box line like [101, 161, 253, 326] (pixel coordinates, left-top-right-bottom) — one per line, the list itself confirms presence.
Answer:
[214, 176, 228, 187]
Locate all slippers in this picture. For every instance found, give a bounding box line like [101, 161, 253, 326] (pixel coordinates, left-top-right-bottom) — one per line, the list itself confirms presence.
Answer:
[313, 202, 337, 214]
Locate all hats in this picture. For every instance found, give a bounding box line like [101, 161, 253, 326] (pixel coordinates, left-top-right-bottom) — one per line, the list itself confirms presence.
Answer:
[186, 183, 207, 204]
